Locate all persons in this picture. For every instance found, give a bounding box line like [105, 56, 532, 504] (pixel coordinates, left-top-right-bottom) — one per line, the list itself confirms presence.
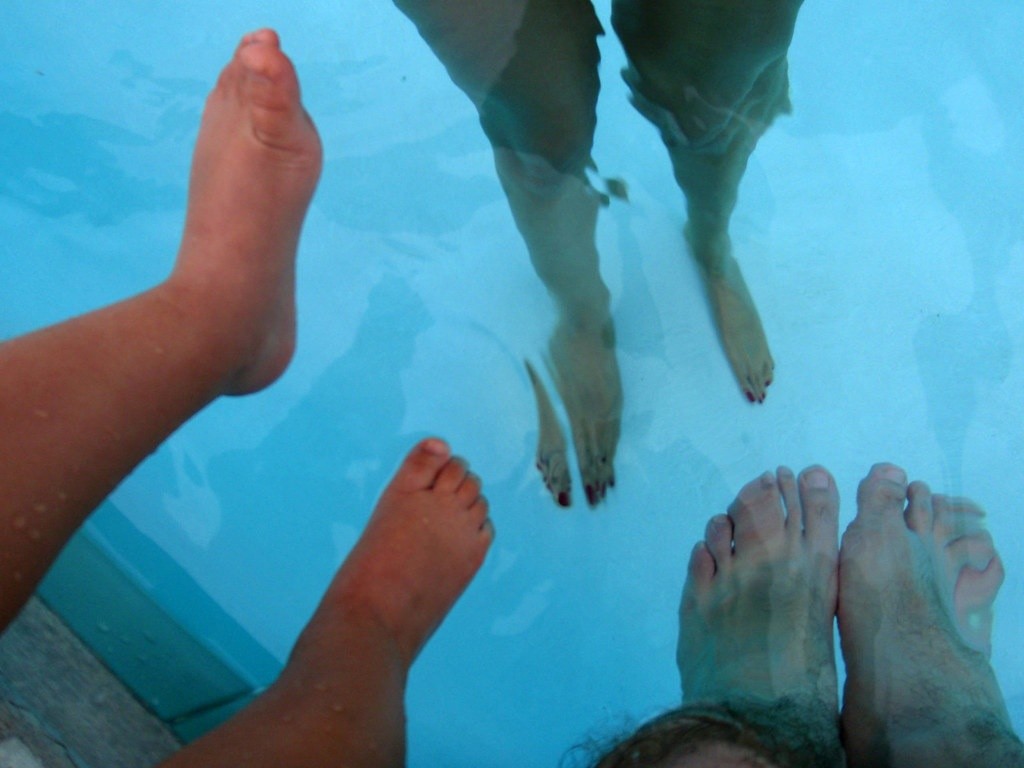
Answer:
[592, 464, 1023, 768]
[399, 1, 802, 507]
[0, 27, 497, 768]
